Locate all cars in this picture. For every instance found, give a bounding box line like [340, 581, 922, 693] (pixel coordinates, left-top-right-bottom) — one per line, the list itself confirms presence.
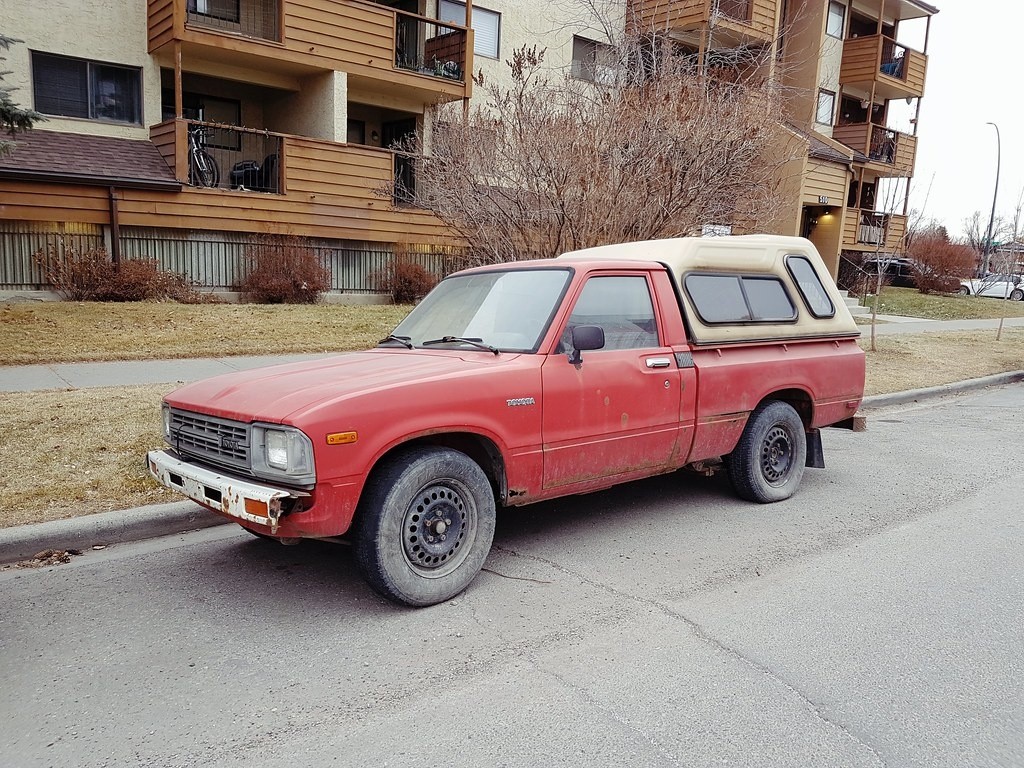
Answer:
[859, 258, 961, 292]
[956, 272, 1024, 302]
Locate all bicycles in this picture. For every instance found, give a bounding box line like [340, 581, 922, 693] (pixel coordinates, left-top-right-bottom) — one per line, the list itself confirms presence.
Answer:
[187, 122, 220, 188]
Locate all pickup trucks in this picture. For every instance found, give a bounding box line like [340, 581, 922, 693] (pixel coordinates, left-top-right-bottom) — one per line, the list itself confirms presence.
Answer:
[144, 233, 867, 607]
